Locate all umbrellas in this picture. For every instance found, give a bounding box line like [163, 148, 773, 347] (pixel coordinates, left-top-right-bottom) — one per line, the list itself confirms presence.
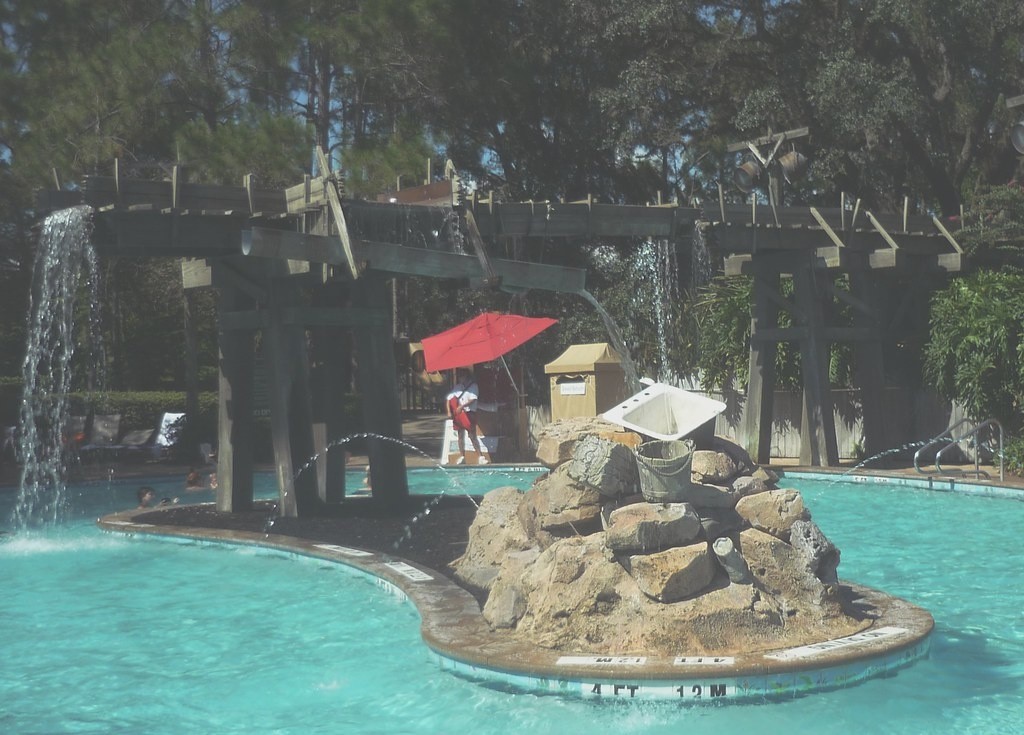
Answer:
[418, 307, 562, 397]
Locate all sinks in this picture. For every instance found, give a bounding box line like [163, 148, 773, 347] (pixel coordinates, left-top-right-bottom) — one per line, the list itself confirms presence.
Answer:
[598, 376, 727, 449]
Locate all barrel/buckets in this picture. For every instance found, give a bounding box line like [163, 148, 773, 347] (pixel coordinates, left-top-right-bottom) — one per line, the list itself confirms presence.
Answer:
[633, 440, 697, 503]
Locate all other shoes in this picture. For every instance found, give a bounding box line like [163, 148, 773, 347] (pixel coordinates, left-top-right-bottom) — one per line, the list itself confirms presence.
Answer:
[457, 456, 466, 465]
[479, 456, 489, 464]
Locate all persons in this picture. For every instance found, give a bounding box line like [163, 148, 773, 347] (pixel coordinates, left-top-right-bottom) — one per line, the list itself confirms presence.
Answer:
[136, 486, 181, 510]
[445, 367, 491, 466]
[183, 470, 218, 493]
